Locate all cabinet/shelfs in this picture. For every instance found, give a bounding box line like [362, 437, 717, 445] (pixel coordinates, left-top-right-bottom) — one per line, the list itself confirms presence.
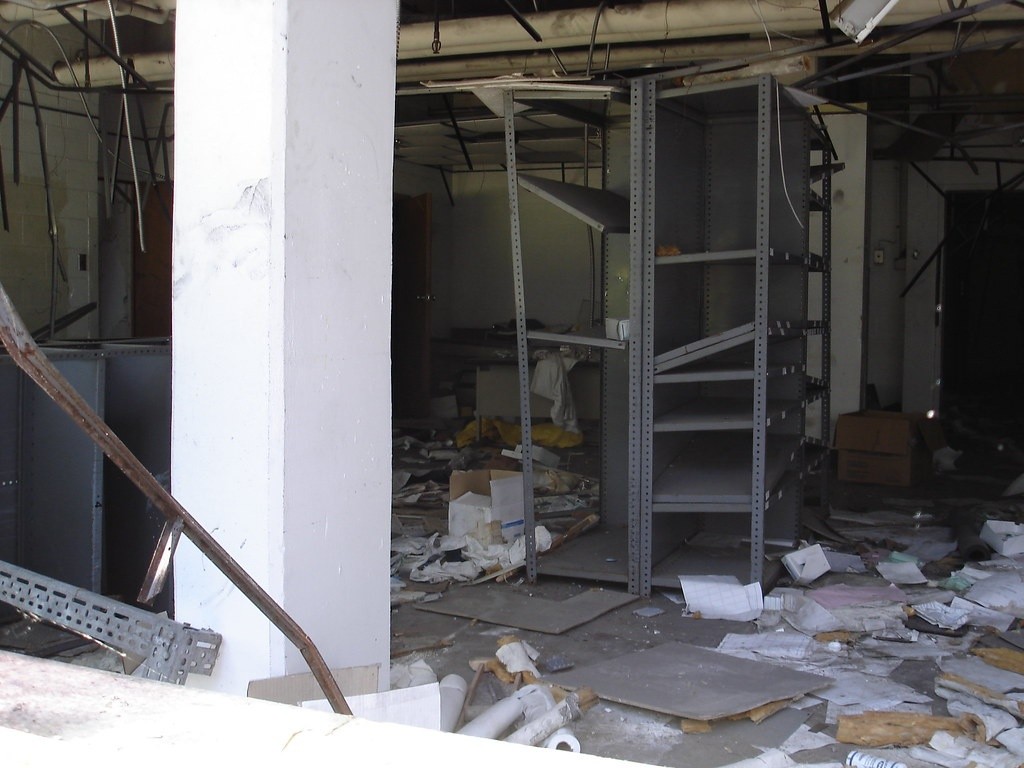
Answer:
[0, 335, 222, 688]
[503, 72, 832, 609]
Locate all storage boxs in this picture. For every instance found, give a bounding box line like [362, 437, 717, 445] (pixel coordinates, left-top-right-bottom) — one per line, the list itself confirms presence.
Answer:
[780, 543, 831, 583]
[450, 467, 525, 553]
[833, 410, 957, 485]
[979, 519, 1024, 556]
